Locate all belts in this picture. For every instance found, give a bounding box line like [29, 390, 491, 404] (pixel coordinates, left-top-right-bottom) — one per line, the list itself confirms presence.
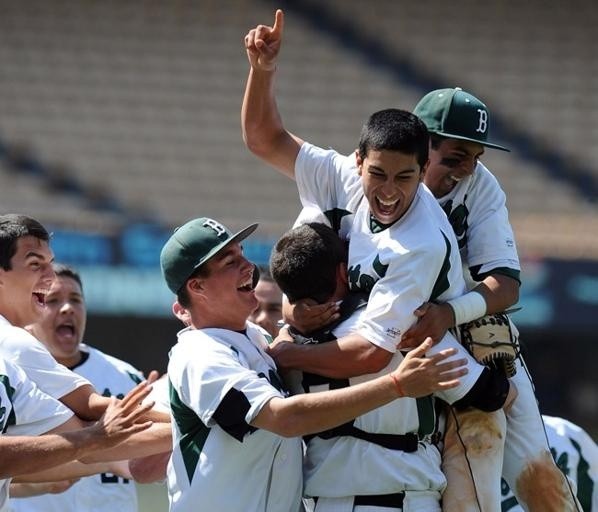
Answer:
[351, 491, 405, 509]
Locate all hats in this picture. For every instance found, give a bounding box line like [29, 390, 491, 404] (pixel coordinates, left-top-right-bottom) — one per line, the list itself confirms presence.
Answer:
[160, 217, 258, 294]
[413, 88, 513, 155]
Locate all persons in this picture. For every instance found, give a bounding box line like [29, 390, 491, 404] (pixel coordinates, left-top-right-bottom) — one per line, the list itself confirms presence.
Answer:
[164, 217, 468, 511]
[0, 379, 158, 477]
[1, 212, 172, 510]
[342, 88, 584, 512]
[29, 264, 172, 509]
[167, 222, 598, 511]
[241, 7, 468, 509]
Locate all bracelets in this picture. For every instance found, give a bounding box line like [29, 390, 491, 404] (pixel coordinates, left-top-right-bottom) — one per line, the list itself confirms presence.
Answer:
[390, 371, 405, 399]
[444, 289, 487, 328]
